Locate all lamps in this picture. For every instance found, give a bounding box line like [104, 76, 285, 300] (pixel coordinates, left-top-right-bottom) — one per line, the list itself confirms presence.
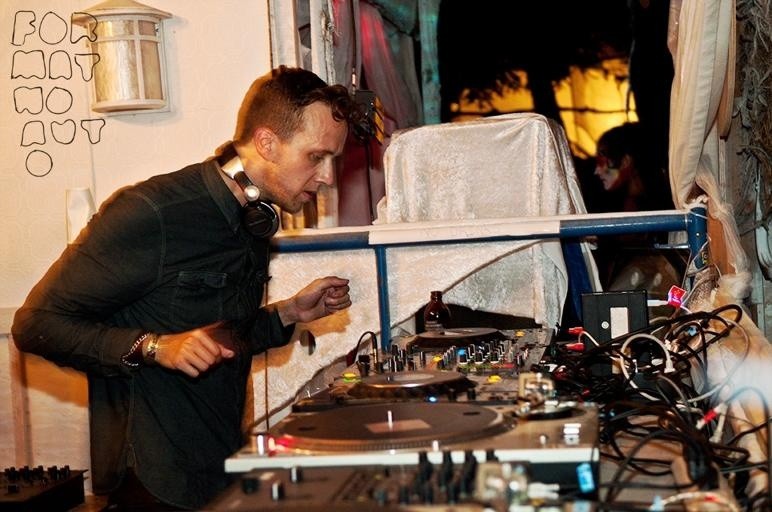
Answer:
[71, 0, 174, 120]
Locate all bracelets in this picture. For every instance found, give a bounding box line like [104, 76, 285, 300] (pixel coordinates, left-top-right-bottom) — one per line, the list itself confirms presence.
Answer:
[144, 332, 161, 363]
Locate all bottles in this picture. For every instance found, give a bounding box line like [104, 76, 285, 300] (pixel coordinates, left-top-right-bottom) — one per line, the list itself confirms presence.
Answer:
[423, 290, 452, 333]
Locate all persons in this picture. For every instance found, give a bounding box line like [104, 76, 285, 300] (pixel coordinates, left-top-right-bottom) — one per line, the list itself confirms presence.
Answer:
[592, 123, 673, 292]
[10, 66, 355, 512]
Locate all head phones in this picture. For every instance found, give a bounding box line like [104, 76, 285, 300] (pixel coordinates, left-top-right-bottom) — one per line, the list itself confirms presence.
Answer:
[214, 139, 280, 242]
